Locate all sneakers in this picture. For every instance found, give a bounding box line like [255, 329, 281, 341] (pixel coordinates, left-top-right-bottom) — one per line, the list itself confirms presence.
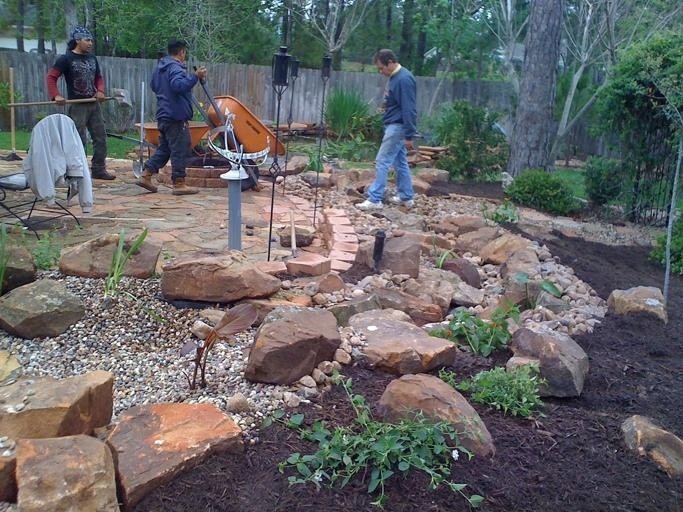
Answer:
[92, 172, 117, 180]
[388, 195, 415, 207]
[355, 200, 384, 211]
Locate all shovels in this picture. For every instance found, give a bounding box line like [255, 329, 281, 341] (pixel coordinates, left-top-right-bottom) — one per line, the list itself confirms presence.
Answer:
[132, 81, 146, 178]
[7, 89, 133, 106]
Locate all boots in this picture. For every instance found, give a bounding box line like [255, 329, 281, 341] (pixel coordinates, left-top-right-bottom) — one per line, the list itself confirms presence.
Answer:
[135, 168, 158, 192]
[172, 176, 199, 195]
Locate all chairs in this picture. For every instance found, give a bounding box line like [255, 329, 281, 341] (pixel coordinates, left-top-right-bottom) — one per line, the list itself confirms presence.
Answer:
[0, 112, 83, 241]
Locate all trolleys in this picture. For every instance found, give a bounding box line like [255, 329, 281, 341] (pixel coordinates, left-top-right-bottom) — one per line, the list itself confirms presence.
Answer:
[106, 122, 211, 160]
[190, 65, 286, 192]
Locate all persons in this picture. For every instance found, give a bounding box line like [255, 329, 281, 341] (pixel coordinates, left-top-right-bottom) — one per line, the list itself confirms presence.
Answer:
[46, 28, 117, 180]
[355, 46, 417, 210]
[134, 38, 207, 195]
